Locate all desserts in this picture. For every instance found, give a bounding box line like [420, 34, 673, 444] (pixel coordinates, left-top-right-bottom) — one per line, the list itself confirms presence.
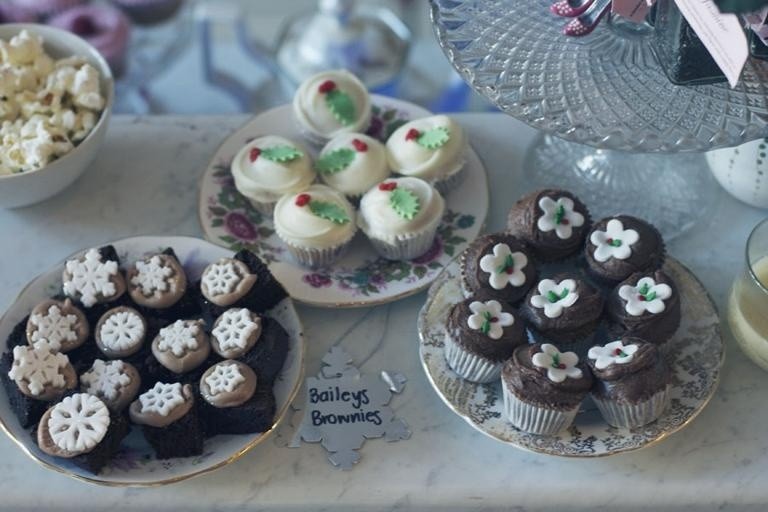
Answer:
[445, 186, 683, 437]
[0, 246, 261, 475]
[232, 70, 465, 268]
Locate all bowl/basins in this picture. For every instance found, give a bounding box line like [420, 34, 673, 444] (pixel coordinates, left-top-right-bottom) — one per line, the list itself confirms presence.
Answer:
[0, 22, 119, 210]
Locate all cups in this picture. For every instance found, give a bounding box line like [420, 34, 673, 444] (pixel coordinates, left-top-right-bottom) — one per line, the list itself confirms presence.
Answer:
[726, 218, 768, 374]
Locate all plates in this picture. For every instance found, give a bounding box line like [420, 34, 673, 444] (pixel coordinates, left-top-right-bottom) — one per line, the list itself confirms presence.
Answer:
[197, 91, 491, 311]
[419, 241, 727, 459]
[1, 235, 307, 486]
